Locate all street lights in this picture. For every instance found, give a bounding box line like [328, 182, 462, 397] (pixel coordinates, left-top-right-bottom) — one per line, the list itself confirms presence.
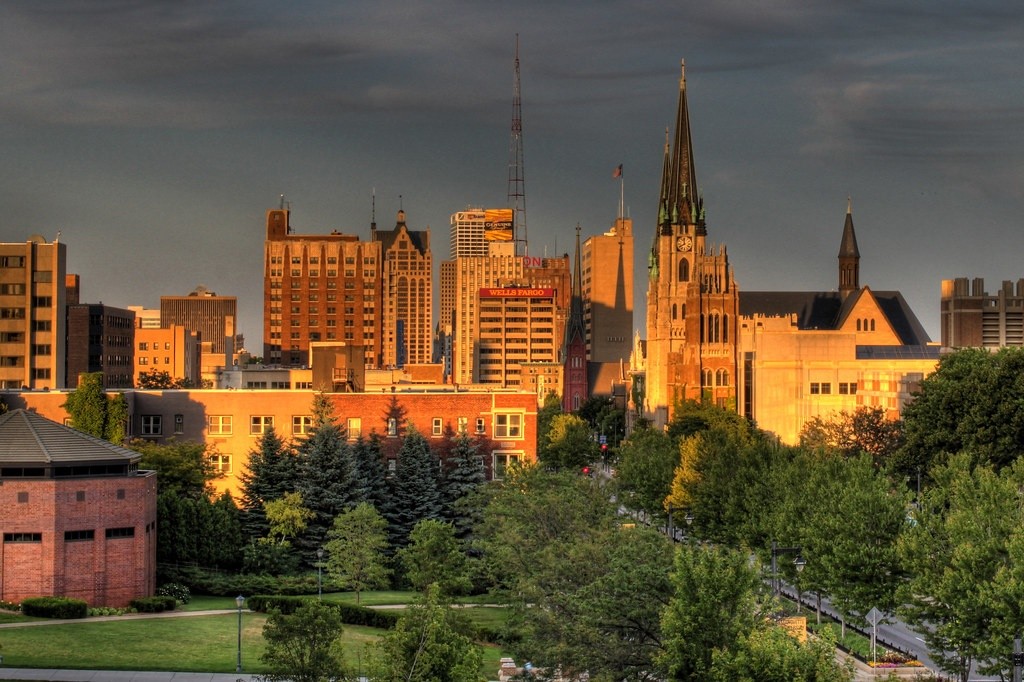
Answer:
[234, 594, 246, 673]
[666, 501, 695, 545]
[609, 418, 624, 448]
[771, 538, 809, 598]
[317, 548, 325, 599]
[916, 464, 924, 511]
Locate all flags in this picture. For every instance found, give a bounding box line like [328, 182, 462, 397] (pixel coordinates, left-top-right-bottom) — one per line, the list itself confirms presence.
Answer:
[612, 164, 622, 177]
